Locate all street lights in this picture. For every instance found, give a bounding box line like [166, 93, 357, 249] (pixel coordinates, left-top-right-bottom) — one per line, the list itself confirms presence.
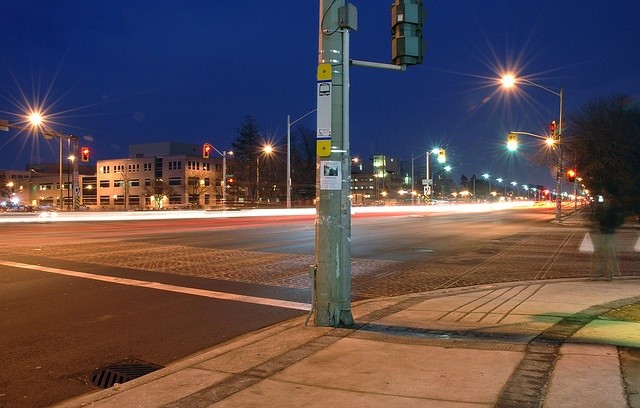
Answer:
[505, 180, 517, 197]
[502, 75, 563, 223]
[473, 173, 490, 199]
[412, 148, 440, 205]
[30, 112, 63, 211]
[489, 177, 502, 197]
[431, 165, 451, 198]
[257, 145, 273, 201]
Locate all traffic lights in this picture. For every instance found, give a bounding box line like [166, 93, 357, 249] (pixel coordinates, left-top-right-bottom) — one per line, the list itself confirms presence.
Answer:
[569, 169, 575, 182]
[203, 144, 210, 158]
[82, 147, 89, 162]
[550, 123, 556, 132]
